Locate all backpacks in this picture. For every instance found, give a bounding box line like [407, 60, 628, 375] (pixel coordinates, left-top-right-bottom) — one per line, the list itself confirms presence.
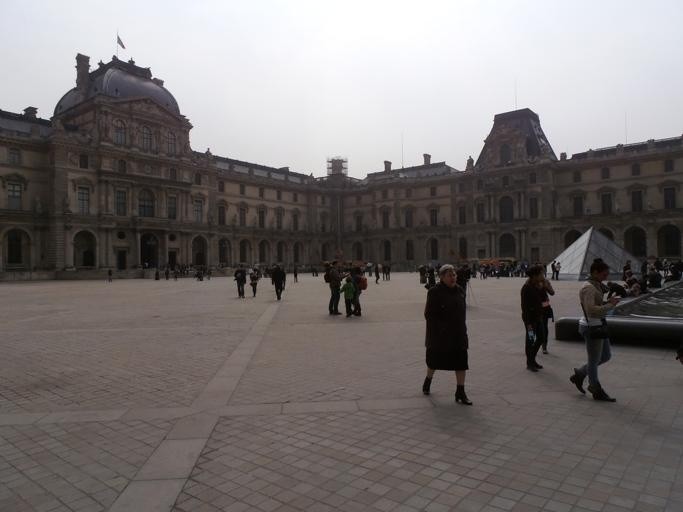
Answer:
[358, 277, 367, 290]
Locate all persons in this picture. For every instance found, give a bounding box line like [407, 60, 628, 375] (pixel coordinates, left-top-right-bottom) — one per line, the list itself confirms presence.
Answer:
[520, 265, 545, 374]
[568, 258, 622, 403]
[423, 261, 474, 406]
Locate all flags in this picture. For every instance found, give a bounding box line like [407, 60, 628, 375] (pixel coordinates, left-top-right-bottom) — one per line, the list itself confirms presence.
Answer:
[117, 35, 125, 48]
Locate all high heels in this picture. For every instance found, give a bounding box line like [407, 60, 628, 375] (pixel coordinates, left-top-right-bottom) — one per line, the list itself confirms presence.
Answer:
[455, 384, 473, 404]
[422, 376, 432, 396]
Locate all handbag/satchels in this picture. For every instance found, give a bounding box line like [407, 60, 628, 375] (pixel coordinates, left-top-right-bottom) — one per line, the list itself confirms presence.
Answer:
[545, 305, 554, 322]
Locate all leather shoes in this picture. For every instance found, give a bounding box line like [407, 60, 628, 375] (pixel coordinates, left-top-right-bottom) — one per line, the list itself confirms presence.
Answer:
[570, 374, 586, 394]
[527, 359, 543, 372]
[593, 390, 617, 402]
